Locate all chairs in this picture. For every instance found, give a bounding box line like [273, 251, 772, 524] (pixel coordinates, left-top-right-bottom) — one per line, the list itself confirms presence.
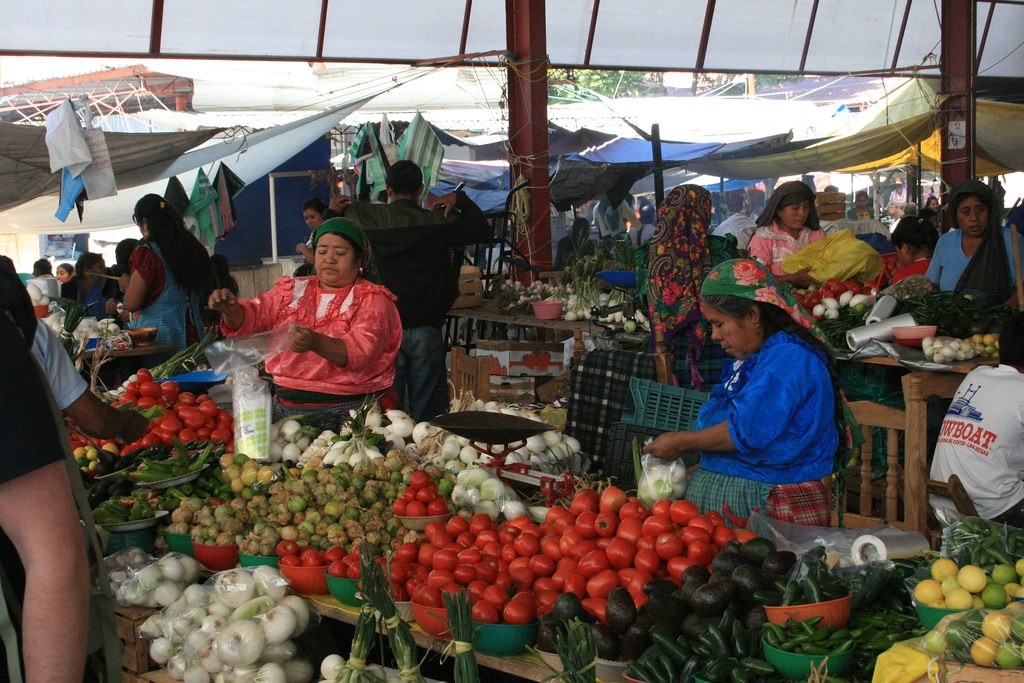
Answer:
[894, 462, 981, 551]
[449, 346, 491, 404]
[830, 374, 928, 537]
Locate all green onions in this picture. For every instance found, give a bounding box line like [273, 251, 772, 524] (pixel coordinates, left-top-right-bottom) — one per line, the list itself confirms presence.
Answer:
[334, 553, 424, 683]
[526, 616, 597, 683]
[57, 300, 84, 362]
[425, 588, 480, 683]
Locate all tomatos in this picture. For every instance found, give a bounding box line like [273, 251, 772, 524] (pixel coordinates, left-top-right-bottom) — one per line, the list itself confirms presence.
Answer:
[792, 279, 875, 323]
[275, 471, 756, 625]
[64, 369, 235, 454]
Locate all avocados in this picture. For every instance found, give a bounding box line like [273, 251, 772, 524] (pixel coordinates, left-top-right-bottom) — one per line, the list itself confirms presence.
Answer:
[538, 536, 797, 661]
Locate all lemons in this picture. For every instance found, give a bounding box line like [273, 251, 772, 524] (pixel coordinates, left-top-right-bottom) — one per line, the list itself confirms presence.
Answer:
[971, 335, 999, 358]
[220, 453, 273, 503]
[915, 559, 1024, 667]
[74, 443, 118, 473]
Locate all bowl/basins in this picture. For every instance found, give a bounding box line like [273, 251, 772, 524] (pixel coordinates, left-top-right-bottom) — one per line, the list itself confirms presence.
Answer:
[763, 595, 853, 628]
[760, 637, 861, 680]
[915, 597, 972, 632]
[390, 601, 415, 625]
[465, 619, 540, 657]
[33, 305, 48, 318]
[239, 552, 280, 573]
[393, 515, 449, 530]
[190, 540, 239, 573]
[128, 327, 158, 347]
[324, 570, 364, 607]
[530, 300, 564, 320]
[412, 601, 455, 638]
[277, 558, 331, 596]
[162, 530, 194, 560]
[892, 326, 936, 348]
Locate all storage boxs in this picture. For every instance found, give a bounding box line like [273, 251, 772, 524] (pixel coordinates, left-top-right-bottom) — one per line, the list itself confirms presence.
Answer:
[814, 192, 848, 221]
[476, 339, 572, 405]
[114, 606, 185, 683]
[451, 266, 483, 308]
[602, 377, 710, 490]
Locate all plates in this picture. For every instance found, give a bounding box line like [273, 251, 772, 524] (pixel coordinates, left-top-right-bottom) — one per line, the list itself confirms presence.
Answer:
[94, 463, 134, 481]
[137, 464, 209, 488]
[95, 510, 168, 531]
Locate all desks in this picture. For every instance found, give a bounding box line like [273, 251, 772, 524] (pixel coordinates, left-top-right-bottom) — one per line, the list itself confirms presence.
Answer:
[443, 298, 610, 351]
[264, 255, 304, 279]
[836, 353, 999, 399]
[83, 345, 175, 359]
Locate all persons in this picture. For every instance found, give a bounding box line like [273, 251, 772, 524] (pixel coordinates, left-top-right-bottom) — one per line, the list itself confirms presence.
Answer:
[208, 215, 403, 433]
[635, 184, 737, 389]
[294, 196, 328, 269]
[32, 258, 54, 280]
[686, 179, 1024, 314]
[642, 256, 862, 528]
[0, 255, 150, 683]
[926, 311, 1024, 530]
[77, 193, 239, 373]
[56, 263, 78, 301]
[553, 189, 656, 273]
[322, 158, 492, 423]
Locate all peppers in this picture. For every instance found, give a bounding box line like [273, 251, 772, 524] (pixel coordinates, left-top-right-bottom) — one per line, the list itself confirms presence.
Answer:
[91, 438, 226, 524]
[946, 517, 1024, 572]
[623, 558, 934, 683]
[818, 291, 1010, 349]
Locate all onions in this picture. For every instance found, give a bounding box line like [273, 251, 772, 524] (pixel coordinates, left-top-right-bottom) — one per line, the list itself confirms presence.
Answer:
[148, 330, 218, 380]
[923, 336, 974, 363]
[104, 548, 345, 683]
[822, 297, 839, 309]
[26, 284, 119, 339]
[839, 290, 853, 307]
[850, 293, 868, 307]
[641, 465, 686, 499]
[269, 399, 580, 520]
[826, 308, 839, 319]
[501, 279, 627, 323]
[813, 304, 825, 318]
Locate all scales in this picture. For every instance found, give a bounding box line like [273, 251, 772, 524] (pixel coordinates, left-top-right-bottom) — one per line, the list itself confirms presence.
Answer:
[431, 409, 583, 502]
[153, 372, 229, 397]
[591, 268, 653, 353]
